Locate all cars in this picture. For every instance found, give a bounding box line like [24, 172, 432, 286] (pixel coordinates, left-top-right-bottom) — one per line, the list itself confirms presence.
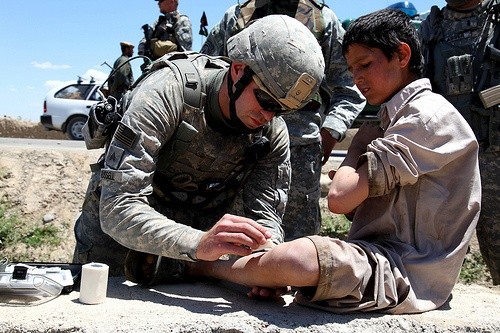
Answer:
[40, 80, 108, 141]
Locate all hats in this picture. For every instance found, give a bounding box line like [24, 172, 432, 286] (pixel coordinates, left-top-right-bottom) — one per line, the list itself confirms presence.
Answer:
[119, 41, 135, 48]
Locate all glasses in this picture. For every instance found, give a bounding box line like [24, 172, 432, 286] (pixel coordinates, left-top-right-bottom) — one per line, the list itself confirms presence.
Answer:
[252, 87, 292, 119]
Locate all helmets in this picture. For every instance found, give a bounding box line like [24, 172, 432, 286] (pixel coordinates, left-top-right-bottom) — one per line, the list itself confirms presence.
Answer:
[227, 14, 328, 112]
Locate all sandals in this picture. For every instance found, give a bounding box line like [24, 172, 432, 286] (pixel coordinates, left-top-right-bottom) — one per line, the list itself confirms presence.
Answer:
[124, 249, 186, 286]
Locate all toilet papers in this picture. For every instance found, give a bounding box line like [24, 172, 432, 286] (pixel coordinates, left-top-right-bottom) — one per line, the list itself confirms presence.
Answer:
[79, 262, 109, 305]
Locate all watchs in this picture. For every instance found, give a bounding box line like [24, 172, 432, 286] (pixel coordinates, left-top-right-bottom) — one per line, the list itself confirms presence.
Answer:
[324, 127, 341, 140]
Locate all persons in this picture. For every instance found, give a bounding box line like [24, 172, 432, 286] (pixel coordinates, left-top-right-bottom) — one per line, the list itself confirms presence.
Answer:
[136, 0, 193, 74]
[414, 0, 500, 287]
[107, 40, 135, 103]
[124, 7, 480, 315]
[71, 14, 326, 301]
[199, 0, 367, 243]
